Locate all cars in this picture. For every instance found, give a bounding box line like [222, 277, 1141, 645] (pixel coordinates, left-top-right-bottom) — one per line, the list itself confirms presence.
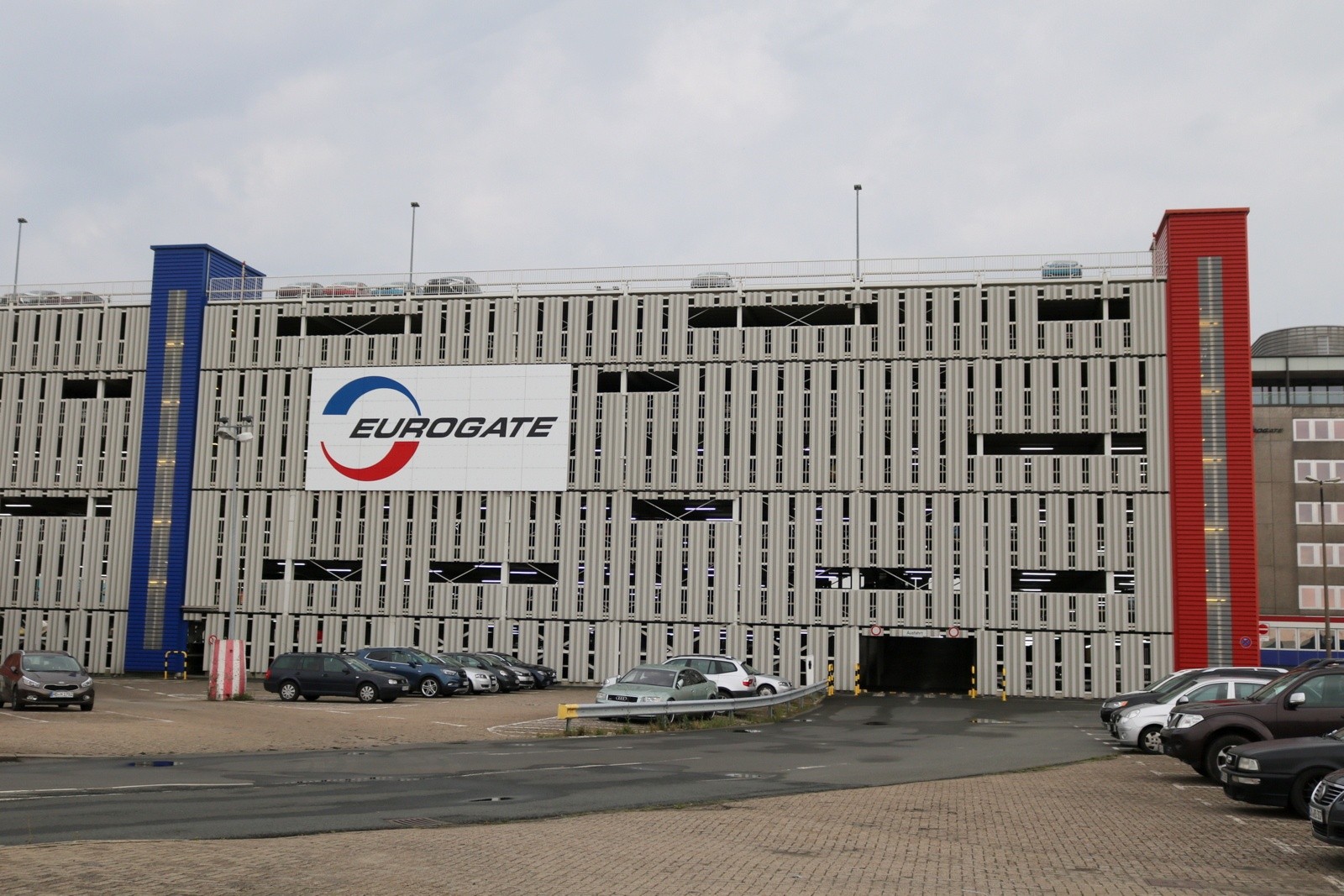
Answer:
[595, 664, 720, 725]
[0, 650, 95, 711]
[1308, 768, 1344, 847]
[1041, 260, 1082, 278]
[275, 281, 325, 298]
[425, 651, 556, 695]
[1220, 724, 1344, 821]
[370, 281, 424, 295]
[423, 275, 483, 294]
[322, 281, 371, 297]
[691, 271, 733, 288]
[0, 289, 104, 311]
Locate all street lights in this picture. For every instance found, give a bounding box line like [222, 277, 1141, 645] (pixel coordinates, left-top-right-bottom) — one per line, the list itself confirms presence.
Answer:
[1304, 475, 1344, 658]
[13, 217, 28, 299]
[854, 183, 861, 280]
[411, 202, 421, 283]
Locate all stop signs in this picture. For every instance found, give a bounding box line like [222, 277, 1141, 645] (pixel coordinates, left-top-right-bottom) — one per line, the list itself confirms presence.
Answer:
[1257, 624, 1269, 635]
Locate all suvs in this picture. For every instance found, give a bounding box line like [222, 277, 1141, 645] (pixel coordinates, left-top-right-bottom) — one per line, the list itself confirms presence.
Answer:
[347, 646, 470, 698]
[600, 653, 793, 717]
[263, 653, 411, 704]
[1100, 663, 1322, 755]
[1159, 656, 1344, 787]
